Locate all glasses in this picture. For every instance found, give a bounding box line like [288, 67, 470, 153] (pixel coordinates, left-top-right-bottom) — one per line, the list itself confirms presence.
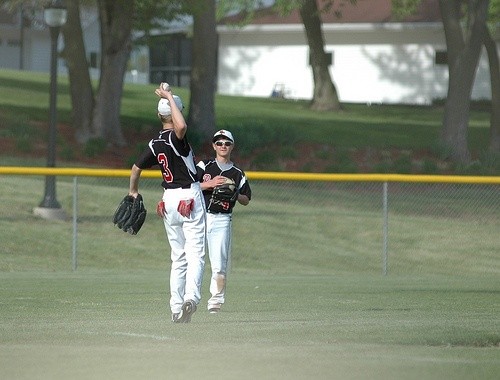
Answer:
[215, 142, 233, 146]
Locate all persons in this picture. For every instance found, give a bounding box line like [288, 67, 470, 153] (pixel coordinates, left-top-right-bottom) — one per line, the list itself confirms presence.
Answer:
[196, 130, 252, 314]
[113, 84, 206, 323]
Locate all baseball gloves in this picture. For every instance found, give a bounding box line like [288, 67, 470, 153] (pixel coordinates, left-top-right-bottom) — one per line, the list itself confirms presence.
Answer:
[211, 176, 237, 201]
[112, 193, 147, 235]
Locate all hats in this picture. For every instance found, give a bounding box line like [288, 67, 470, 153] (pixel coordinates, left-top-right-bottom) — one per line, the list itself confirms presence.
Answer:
[158, 95, 183, 116]
[213, 129, 234, 142]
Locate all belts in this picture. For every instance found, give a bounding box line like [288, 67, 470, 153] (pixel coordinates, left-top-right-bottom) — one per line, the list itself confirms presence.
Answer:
[182, 184, 191, 189]
[206, 209, 228, 214]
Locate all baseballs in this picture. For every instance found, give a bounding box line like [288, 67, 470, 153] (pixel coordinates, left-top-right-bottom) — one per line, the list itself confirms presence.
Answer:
[162, 82, 169, 90]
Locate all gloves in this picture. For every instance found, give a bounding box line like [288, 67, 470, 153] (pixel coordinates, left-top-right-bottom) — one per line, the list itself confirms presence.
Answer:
[178, 199, 194, 219]
[157, 202, 167, 217]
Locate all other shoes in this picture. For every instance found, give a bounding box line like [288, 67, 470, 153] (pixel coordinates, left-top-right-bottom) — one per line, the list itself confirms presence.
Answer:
[172, 313, 180, 323]
[210, 308, 220, 313]
[176, 300, 196, 323]
